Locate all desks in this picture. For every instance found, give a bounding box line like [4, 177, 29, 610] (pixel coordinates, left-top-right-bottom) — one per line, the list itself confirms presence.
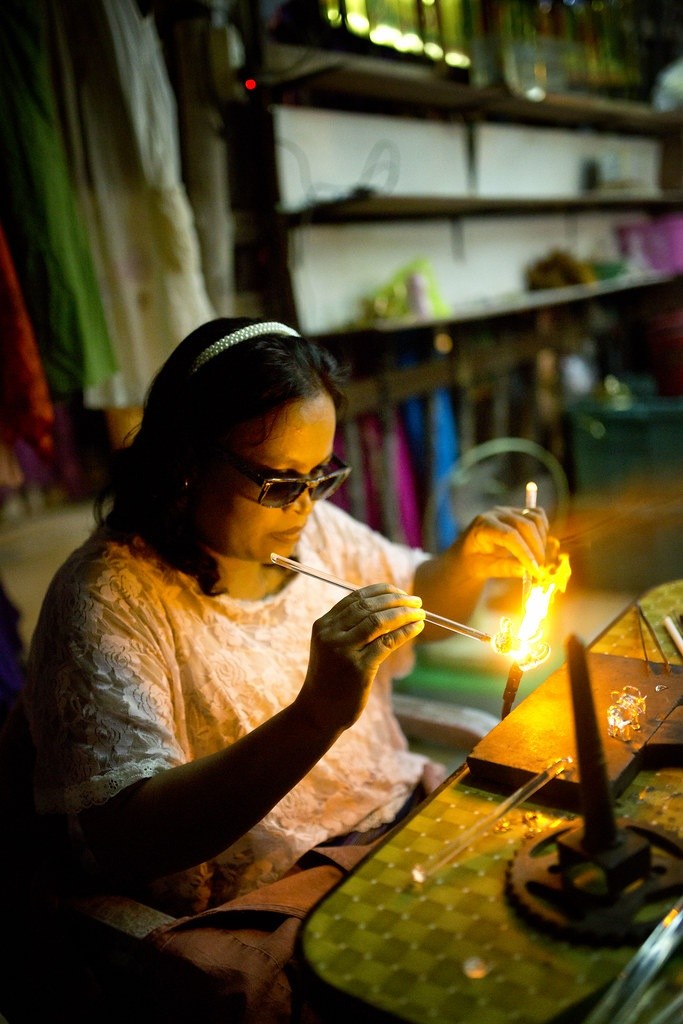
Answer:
[294, 578, 683, 1024]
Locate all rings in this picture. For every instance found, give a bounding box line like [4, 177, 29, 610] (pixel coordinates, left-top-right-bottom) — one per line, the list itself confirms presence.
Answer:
[520, 507, 533, 515]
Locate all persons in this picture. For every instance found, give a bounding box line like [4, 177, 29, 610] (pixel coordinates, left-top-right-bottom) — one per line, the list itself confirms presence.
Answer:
[17, 318, 552, 1023]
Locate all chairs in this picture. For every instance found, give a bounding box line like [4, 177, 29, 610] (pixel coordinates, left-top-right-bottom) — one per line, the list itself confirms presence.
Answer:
[0, 505, 502, 979]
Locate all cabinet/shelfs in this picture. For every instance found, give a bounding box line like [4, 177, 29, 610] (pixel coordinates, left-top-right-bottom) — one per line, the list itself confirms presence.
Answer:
[220, 0, 683, 346]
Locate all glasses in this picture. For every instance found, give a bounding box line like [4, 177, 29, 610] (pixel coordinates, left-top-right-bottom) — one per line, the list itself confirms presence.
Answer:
[218, 447, 353, 510]
